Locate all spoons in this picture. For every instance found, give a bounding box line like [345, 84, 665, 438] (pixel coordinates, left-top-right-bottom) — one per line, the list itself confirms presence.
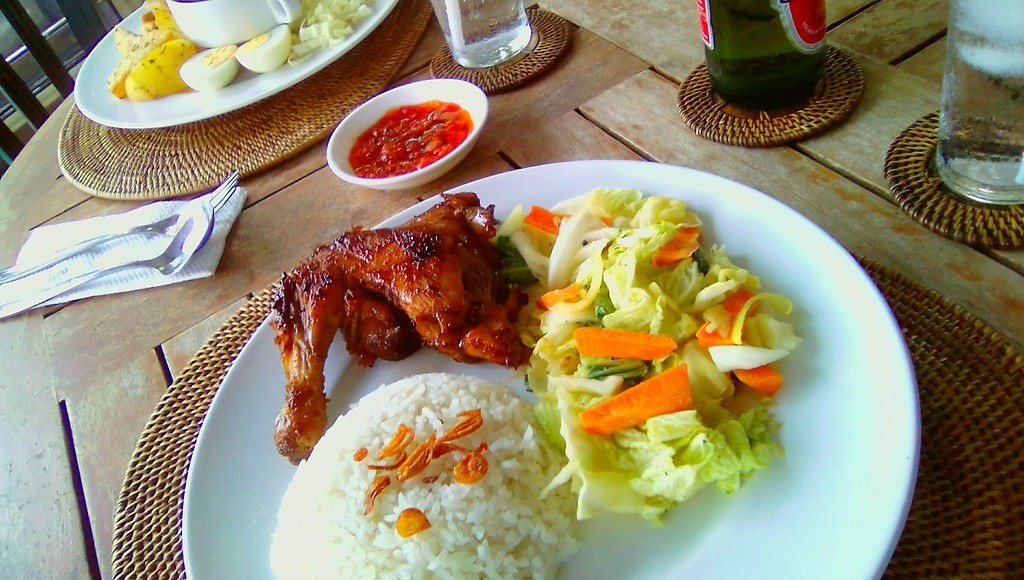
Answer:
[0, 203, 218, 320]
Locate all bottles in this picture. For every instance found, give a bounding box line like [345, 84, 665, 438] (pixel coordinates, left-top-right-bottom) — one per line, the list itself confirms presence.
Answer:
[693, 0, 828, 116]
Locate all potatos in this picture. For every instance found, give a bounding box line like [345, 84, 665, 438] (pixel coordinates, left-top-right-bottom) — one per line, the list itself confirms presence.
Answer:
[124, 37, 196, 101]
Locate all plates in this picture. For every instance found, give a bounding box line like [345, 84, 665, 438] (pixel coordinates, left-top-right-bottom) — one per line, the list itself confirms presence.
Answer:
[73, 0, 404, 130]
[181, 160, 923, 580]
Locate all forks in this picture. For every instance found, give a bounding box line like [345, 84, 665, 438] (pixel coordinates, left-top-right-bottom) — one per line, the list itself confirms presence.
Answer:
[0, 169, 240, 285]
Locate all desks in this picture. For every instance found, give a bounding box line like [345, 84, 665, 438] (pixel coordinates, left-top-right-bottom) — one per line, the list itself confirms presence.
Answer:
[0, 0, 1024, 580]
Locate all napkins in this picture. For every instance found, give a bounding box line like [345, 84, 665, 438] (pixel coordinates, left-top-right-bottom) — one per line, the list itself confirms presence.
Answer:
[0, 186, 249, 315]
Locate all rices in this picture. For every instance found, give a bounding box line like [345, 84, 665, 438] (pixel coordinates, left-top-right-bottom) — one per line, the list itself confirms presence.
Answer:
[268, 371, 579, 580]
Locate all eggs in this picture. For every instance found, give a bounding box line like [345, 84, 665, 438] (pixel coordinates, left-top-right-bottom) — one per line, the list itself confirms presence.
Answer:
[235, 24, 292, 73]
[179, 43, 239, 92]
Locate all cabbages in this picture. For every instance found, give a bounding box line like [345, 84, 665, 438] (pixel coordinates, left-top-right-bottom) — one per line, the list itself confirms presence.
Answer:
[497, 184, 803, 524]
[286, 0, 375, 65]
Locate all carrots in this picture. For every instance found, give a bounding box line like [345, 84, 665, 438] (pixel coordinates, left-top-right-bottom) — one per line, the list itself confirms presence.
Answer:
[524, 205, 611, 236]
[651, 227, 700, 266]
[695, 289, 758, 348]
[572, 327, 678, 361]
[580, 362, 692, 435]
[731, 364, 783, 396]
[534, 283, 579, 310]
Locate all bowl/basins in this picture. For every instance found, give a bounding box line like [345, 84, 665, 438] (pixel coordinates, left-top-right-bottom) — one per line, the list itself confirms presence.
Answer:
[326, 77, 490, 191]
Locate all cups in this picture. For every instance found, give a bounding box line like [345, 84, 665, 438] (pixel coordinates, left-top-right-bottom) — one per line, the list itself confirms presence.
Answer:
[165, 0, 303, 49]
[935, 0, 1024, 211]
[430, 0, 533, 69]
